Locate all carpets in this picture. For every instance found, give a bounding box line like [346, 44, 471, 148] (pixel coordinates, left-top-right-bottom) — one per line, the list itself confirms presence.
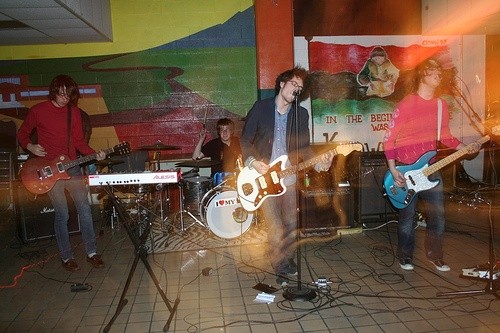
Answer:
[144, 215, 267, 254]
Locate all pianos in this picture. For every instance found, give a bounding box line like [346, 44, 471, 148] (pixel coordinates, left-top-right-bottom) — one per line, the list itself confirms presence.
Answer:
[87, 168, 181, 333]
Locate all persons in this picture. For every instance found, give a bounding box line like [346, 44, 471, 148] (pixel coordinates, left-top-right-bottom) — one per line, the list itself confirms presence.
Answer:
[241, 67, 334, 286]
[17, 75, 107, 270]
[382, 58, 481, 272]
[193, 119, 243, 185]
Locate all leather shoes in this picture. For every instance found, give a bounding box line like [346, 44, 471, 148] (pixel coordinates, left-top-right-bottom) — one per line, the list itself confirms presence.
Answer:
[61, 258, 81, 272]
[87, 254, 105, 269]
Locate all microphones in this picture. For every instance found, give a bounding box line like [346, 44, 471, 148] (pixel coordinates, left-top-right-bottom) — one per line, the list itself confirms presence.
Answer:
[292, 91, 299, 97]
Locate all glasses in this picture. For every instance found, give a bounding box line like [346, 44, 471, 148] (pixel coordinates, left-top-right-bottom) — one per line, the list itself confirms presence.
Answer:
[286, 80, 303, 91]
[424, 67, 442, 71]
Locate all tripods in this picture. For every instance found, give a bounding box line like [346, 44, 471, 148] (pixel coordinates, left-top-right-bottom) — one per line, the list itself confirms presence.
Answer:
[165, 182, 206, 233]
[436, 77, 500, 299]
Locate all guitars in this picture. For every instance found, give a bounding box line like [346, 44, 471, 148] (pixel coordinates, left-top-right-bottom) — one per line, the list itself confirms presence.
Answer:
[384, 125, 500, 210]
[20, 141, 131, 195]
[236, 141, 362, 212]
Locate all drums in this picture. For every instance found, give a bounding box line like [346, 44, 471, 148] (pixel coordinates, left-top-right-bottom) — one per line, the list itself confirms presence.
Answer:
[183, 176, 214, 215]
[121, 184, 158, 192]
[199, 185, 255, 240]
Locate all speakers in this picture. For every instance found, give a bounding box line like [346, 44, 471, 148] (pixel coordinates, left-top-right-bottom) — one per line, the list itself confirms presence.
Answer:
[11, 181, 81, 242]
[300, 150, 403, 229]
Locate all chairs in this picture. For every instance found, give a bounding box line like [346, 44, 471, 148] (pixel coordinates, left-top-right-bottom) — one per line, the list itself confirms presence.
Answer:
[0, 118, 19, 209]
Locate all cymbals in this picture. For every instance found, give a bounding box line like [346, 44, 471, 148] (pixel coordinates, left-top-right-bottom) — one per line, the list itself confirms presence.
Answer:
[174, 160, 221, 167]
[134, 143, 182, 150]
[93, 159, 123, 166]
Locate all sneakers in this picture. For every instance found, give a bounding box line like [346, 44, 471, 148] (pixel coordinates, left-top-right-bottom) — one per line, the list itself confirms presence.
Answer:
[287, 267, 298, 276]
[398, 258, 414, 270]
[276, 277, 288, 285]
[428, 256, 450, 271]
